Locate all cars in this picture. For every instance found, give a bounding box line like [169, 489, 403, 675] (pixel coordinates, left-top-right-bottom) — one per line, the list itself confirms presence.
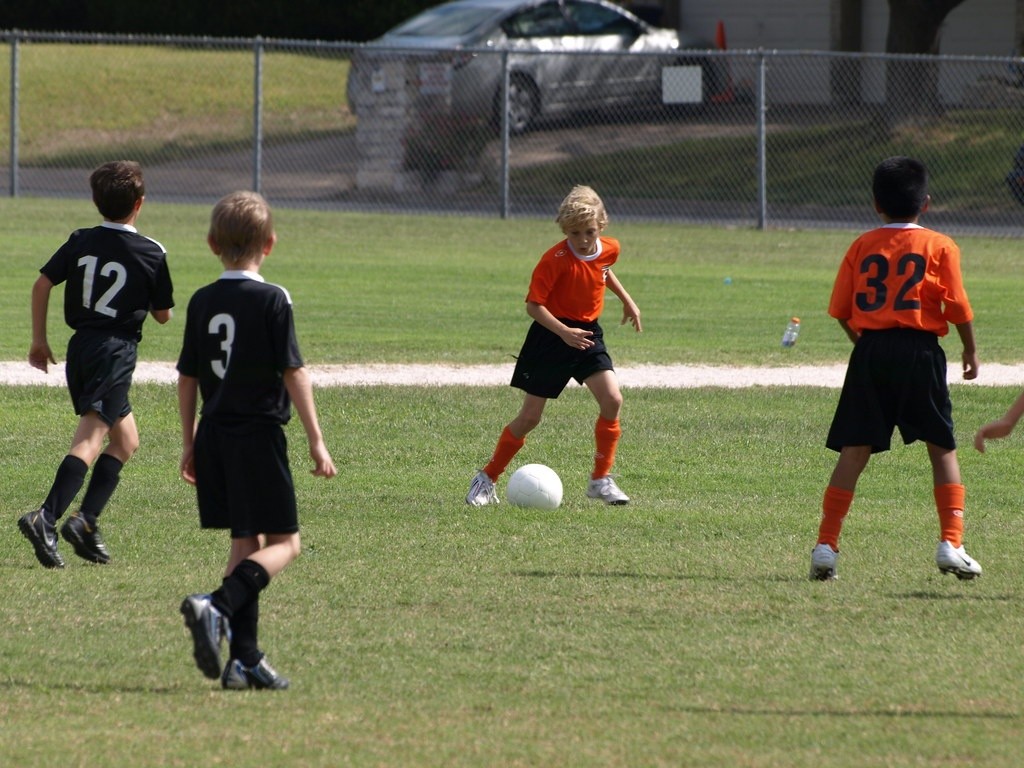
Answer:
[346, 0, 733, 139]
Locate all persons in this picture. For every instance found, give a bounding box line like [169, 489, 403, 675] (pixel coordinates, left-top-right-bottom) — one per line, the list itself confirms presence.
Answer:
[177, 190, 338, 690]
[15, 161, 177, 572]
[465, 185, 643, 511]
[975, 391, 1024, 456]
[808, 155, 983, 583]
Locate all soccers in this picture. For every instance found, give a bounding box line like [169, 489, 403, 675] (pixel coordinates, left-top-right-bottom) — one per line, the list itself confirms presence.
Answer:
[505, 463, 563, 510]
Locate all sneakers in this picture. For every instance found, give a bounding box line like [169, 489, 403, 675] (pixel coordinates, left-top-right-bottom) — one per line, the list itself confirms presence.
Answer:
[938, 539, 983, 579]
[587, 475, 630, 505]
[181, 594, 232, 679]
[222, 652, 289, 689]
[60, 511, 111, 564]
[17, 507, 64, 568]
[466, 471, 499, 507]
[809, 543, 839, 582]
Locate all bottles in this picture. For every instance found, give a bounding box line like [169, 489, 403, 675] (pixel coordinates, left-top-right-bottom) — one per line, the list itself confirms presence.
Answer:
[781, 317, 800, 348]
